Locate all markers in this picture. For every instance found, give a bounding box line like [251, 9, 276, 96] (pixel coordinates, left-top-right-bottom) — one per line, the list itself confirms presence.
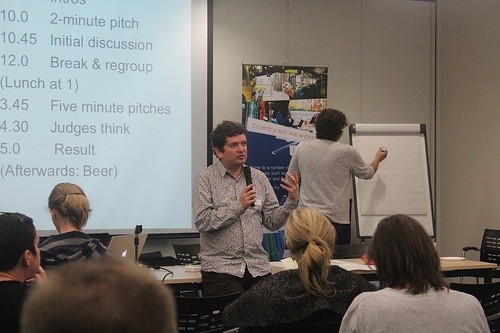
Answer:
[381, 148, 386, 152]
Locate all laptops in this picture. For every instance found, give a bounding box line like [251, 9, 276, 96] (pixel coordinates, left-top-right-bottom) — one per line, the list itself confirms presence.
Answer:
[107, 232, 148, 263]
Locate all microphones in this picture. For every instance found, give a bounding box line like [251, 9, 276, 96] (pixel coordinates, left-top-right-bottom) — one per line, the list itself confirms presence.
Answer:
[243, 165, 255, 208]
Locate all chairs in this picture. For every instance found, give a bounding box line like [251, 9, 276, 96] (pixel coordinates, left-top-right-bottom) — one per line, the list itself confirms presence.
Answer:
[449, 227, 500, 333]
[138, 243, 243, 333]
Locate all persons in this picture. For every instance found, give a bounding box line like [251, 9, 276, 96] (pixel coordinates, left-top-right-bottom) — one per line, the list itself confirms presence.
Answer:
[282, 82, 318, 129]
[22, 256, 175, 333]
[287, 108, 388, 290]
[193, 121, 299, 331]
[340, 214, 491, 333]
[0, 212, 47, 333]
[24, 183, 113, 287]
[223, 207, 378, 333]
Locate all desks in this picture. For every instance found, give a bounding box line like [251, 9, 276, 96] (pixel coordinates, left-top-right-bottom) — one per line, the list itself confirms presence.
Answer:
[156, 255, 497, 286]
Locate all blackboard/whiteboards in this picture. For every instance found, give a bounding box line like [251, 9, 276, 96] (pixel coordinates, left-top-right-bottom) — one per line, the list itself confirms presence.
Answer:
[348, 124, 436, 239]
